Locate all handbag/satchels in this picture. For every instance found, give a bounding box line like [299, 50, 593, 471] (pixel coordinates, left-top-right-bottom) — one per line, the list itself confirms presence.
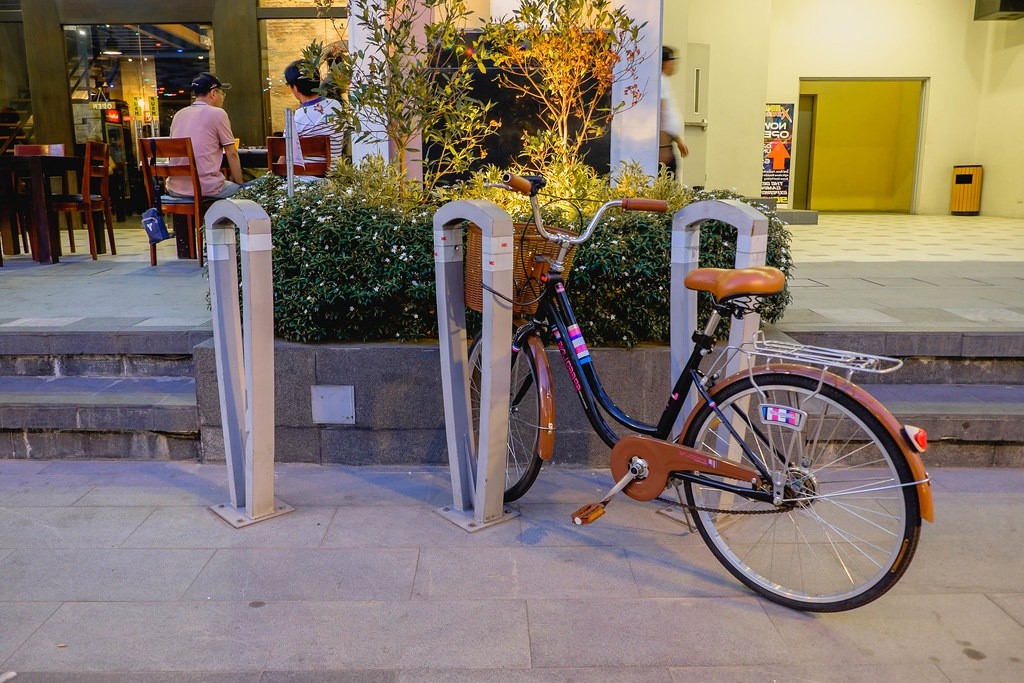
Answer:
[141, 207, 170, 246]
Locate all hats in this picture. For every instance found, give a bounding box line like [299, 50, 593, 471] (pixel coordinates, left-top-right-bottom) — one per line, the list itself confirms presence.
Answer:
[191, 72, 233, 91]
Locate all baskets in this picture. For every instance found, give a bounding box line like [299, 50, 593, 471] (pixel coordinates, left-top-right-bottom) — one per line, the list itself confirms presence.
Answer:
[466, 221, 580, 317]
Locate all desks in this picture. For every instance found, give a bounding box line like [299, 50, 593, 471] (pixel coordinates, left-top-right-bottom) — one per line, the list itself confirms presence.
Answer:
[0, 156, 107, 265]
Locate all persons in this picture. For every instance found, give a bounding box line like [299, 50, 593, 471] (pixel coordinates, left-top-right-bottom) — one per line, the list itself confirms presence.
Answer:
[321, 47, 347, 108]
[238, 58, 345, 199]
[164, 72, 241, 199]
[108, 156, 116, 175]
[659, 46, 690, 158]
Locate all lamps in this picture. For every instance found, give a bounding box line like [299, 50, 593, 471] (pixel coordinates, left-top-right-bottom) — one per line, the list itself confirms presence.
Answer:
[99, 26, 123, 55]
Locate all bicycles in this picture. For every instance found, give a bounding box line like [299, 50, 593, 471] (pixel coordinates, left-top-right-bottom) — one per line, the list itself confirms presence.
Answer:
[465, 173, 935, 614]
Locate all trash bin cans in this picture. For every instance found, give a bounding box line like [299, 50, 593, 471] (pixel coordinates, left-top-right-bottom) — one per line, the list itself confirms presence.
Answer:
[949, 164, 984, 216]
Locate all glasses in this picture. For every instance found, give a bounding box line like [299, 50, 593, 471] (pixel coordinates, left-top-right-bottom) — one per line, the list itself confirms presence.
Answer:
[285, 83, 290, 87]
[216, 89, 226, 97]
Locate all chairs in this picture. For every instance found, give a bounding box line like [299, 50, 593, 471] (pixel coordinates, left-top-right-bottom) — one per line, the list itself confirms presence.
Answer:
[266, 134, 331, 175]
[12, 144, 76, 254]
[0, 191, 36, 266]
[139, 136, 217, 267]
[52, 141, 117, 260]
